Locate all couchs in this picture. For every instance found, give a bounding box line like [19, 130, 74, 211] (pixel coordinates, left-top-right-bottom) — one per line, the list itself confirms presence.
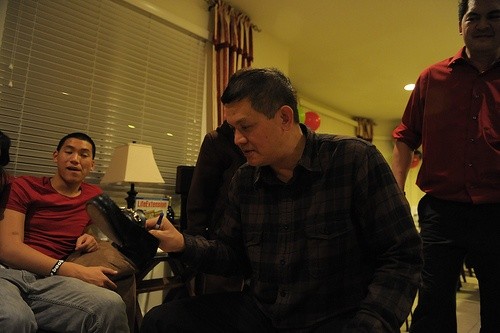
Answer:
[64, 228, 138, 333]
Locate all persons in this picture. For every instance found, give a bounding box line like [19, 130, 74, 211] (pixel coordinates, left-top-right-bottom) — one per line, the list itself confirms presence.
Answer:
[142, 69, 425, 333]
[0, 132, 130, 333]
[0, 131, 11, 221]
[186, 119, 248, 254]
[390, 0, 500, 333]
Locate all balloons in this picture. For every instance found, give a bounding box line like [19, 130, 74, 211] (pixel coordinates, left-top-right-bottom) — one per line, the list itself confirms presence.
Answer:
[305, 111, 320, 131]
[296, 106, 306, 124]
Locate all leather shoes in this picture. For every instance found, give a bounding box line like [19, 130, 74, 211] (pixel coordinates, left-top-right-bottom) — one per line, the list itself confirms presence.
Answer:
[86, 193, 159, 272]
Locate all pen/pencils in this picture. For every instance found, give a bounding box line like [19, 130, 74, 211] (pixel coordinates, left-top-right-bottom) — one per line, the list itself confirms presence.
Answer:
[153, 213, 164, 231]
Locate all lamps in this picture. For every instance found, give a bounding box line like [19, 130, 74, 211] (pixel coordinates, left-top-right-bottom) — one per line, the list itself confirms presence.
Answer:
[101, 140, 165, 209]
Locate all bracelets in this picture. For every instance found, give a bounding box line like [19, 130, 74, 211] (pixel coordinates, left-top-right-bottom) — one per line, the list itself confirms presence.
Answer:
[50, 259, 64, 276]
